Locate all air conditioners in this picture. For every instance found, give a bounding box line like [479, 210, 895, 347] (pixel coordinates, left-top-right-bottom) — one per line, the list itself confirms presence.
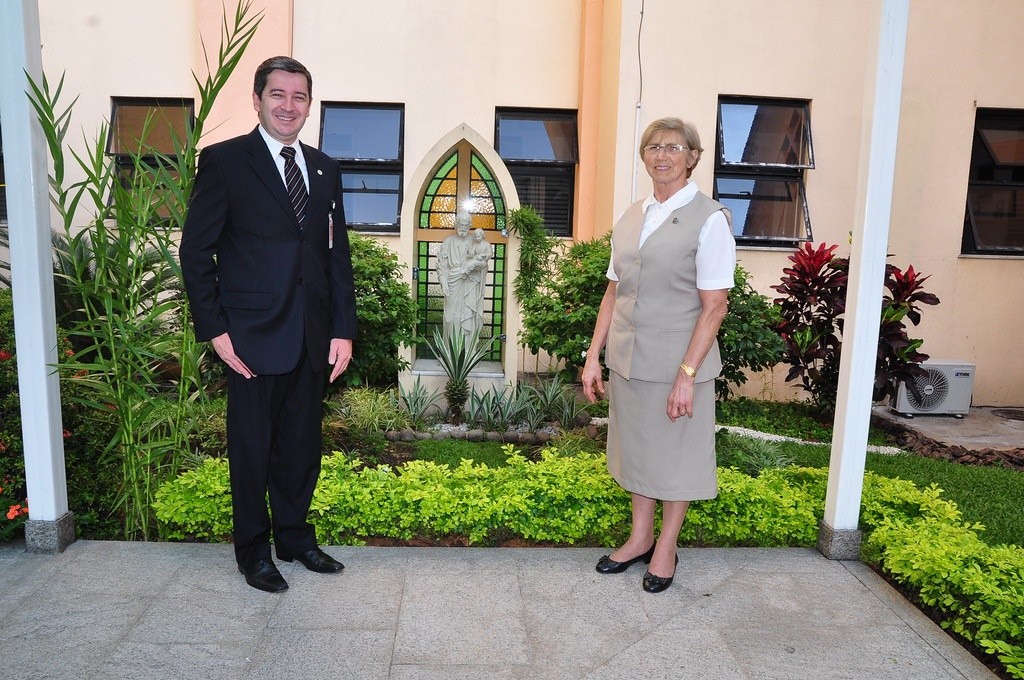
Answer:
[888, 361, 977, 421]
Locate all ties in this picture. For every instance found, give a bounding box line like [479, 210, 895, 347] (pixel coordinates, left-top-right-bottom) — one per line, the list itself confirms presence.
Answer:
[279, 146, 310, 229]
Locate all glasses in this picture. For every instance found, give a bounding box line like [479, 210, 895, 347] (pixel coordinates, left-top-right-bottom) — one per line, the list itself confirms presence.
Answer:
[642, 143, 689, 155]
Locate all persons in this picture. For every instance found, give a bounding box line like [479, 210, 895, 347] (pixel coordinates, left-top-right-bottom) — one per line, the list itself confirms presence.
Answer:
[581, 117, 737, 592]
[460, 228, 493, 275]
[438, 213, 493, 357]
[178, 56, 356, 591]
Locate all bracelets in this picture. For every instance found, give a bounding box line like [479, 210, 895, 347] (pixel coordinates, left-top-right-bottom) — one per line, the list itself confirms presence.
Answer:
[681, 364, 696, 377]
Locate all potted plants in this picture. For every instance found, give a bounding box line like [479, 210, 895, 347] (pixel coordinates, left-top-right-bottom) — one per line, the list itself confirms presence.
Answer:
[148, 332, 195, 380]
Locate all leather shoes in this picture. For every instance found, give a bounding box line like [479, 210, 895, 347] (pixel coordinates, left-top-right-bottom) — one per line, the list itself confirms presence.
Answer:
[596, 537, 658, 574]
[237, 559, 289, 594]
[643, 552, 678, 594]
[276, 542, 345, 574]
